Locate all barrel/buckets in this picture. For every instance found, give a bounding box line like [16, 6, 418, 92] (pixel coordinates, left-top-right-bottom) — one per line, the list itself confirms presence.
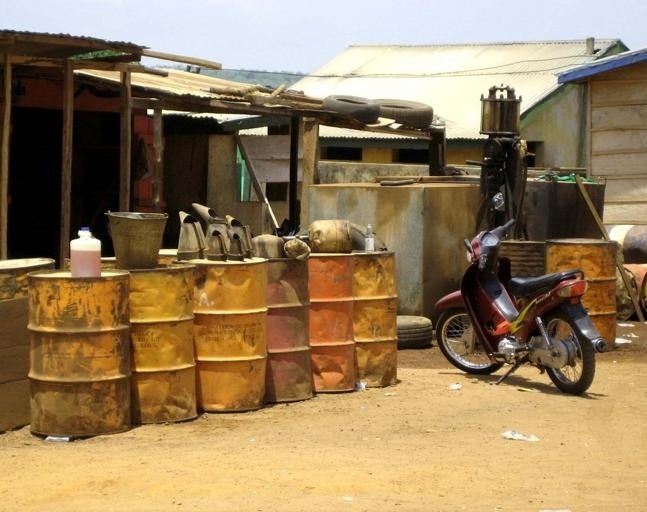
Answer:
[173, 256, 270, 413]
[25, 268, 133, 440]
[352, 250, 400, 389]
[308, 219, 353, 254]
[546, 238, 619, 352]
[1, 259, 57, 300]
[158, 248, 178, 265]
[102, 264, 198, 425]
[496, 240, 546, 278]
[267, 257, 313, 404]
[308, 252, 355, 394]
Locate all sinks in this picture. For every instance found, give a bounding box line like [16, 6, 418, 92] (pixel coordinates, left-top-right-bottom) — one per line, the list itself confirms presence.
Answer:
[105, 209, 168, 270]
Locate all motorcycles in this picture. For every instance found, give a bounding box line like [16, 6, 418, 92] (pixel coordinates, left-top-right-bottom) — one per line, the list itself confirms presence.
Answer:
[435, 218, 609, 396]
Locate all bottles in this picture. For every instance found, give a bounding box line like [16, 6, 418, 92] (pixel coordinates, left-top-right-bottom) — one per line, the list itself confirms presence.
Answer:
[364, 223, 377, 252]
